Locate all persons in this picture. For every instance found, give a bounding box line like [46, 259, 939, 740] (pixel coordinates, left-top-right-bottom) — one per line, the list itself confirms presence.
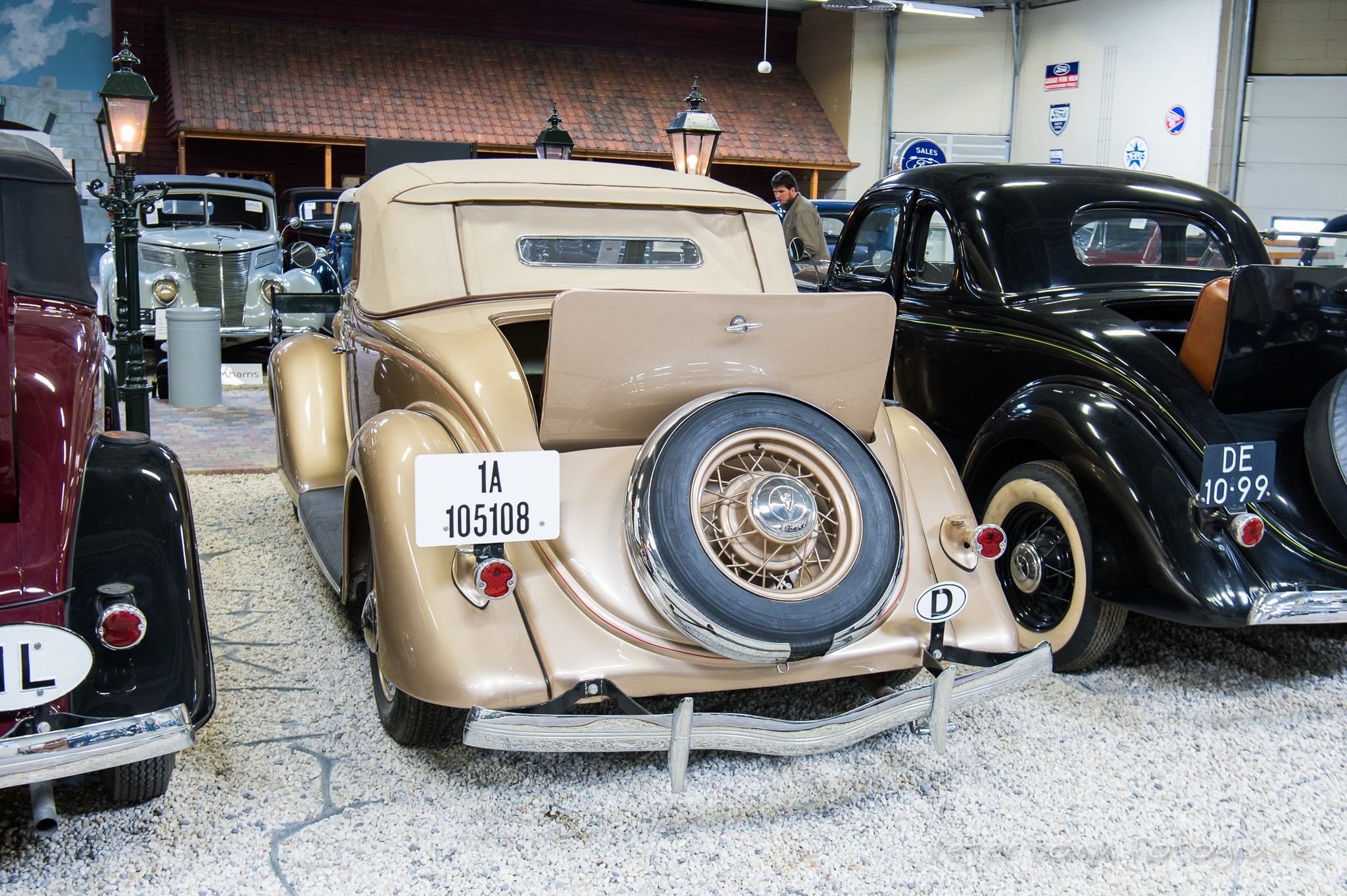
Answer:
[773, 170, 831, 266]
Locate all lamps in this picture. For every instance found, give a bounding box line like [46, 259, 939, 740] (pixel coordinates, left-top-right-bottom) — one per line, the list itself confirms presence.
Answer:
[902, 2, 985, 18]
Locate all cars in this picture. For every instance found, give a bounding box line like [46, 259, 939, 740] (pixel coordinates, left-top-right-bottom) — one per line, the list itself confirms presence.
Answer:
[817, 160, 1347, 670]
[96, 173, 325, 367]
[261, 157, 1054, 798]
[1, 119, 218, 841]
[768, 199, 898, 290]
[272, 185, 346, 293]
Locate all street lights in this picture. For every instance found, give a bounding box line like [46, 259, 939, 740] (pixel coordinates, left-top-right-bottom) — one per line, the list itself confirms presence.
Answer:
[84, 107, 155, 403]
[84, 25, 156, 436]
